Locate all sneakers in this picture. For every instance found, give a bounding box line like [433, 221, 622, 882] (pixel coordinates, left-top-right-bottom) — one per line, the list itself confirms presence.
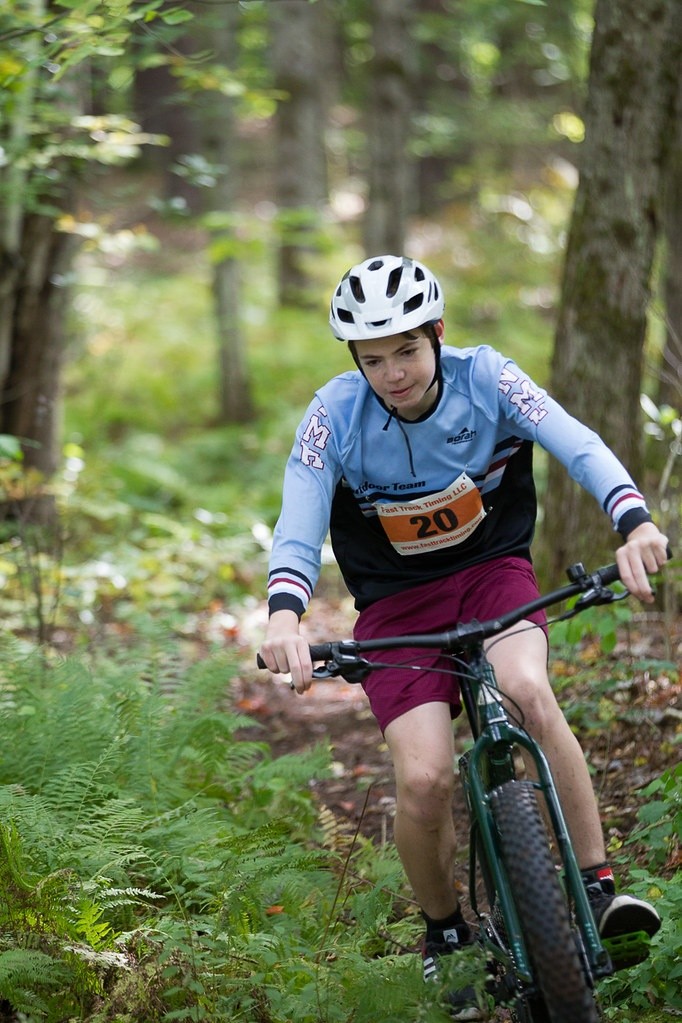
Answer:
[421, 935, 490, 1022]
[561, 864, 662, 969]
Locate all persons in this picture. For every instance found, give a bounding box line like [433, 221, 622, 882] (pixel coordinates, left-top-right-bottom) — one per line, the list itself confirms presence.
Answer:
[260, 254, 671, 1016]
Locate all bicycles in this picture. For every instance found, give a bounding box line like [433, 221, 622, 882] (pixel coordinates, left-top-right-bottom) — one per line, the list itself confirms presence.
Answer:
[257, 548, 678, 1023]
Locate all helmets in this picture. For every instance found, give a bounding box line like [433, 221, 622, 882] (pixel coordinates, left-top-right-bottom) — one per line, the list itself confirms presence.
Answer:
[329, 255, 444, 341]
